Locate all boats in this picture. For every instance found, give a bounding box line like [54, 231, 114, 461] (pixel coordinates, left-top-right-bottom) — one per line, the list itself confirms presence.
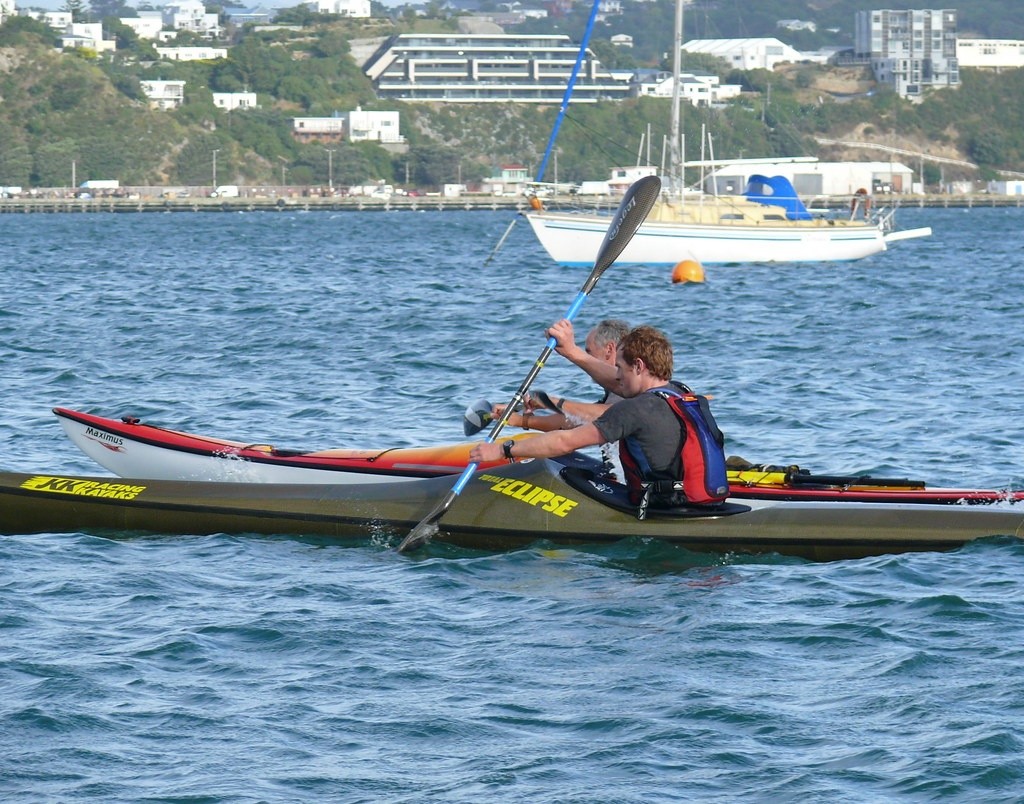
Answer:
[0, 454, 1024, 563]
[53, 405, 1024, 511]
[519, 123, 933, 266]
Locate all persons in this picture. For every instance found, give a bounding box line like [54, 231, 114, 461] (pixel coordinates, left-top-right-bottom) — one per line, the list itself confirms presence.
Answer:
[468, 326, 729, 520]
[492, 320, 632, 460]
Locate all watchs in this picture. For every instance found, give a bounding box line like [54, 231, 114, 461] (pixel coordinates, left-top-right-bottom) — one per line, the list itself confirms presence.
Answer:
[503, 440, 514, 458]
[522, 412, 534, 430]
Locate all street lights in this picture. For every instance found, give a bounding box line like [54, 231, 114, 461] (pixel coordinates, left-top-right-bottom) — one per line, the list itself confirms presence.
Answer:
[208, 149, 219, 186]
[325, 149, 336, 185]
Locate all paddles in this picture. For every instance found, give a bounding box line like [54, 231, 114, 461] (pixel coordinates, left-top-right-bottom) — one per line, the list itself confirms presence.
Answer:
[394, 173, 664, 554]
[463, 390, 566, 437]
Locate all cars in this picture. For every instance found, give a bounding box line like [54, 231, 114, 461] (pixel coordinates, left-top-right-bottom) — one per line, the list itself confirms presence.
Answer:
[0, 180, 422, 199]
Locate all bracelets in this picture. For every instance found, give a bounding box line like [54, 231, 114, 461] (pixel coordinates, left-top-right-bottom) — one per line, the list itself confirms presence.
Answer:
[557, 398, 565, 408]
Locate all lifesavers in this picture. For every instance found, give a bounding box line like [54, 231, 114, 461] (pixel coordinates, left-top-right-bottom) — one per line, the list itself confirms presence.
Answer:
[222, 202, 230, 209]
[851, 187, 870, 217]
[278, 199, 285, 207]
